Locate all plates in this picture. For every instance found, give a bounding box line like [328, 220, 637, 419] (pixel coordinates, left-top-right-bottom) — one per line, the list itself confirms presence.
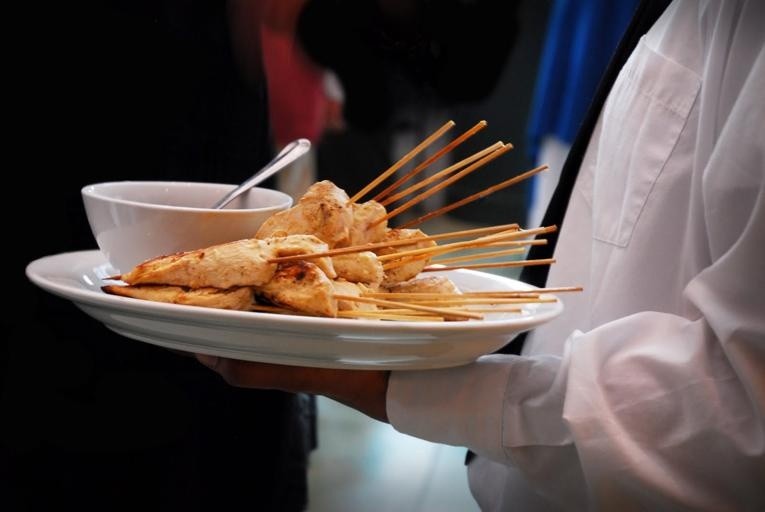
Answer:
[23, 249, 564, 370]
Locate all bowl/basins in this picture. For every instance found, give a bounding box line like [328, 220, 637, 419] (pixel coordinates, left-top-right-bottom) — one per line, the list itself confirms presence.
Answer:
[81, 179, 293, 275]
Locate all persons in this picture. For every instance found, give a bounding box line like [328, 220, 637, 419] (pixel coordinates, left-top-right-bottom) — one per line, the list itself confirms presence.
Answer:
[194, 0, 764, 512]
